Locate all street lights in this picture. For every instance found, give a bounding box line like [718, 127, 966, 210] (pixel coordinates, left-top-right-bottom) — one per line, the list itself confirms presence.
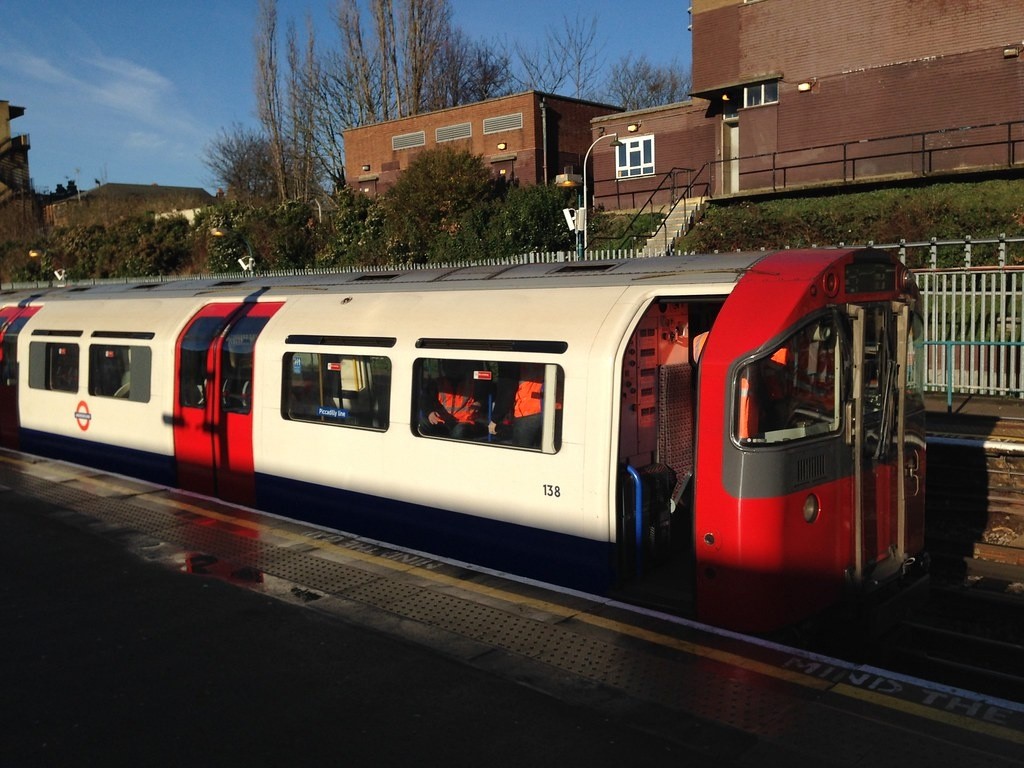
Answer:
[583, 130, 624, 263]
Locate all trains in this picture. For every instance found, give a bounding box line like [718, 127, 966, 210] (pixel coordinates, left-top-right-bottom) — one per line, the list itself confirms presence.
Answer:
[1, 245, 928, 632]
